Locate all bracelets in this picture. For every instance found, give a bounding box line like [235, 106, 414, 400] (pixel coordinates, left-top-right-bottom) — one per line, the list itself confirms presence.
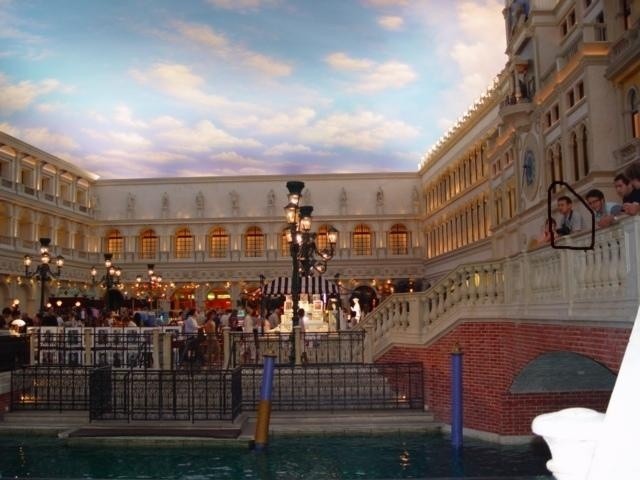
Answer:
[614, 213, 618, 223]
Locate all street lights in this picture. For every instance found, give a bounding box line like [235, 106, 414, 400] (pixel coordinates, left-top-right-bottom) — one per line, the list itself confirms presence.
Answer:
[23, 237, 64, 319]
[282, 181, 339, 370]
[136, 263, 163, 309]
[91, 254, 122, 290]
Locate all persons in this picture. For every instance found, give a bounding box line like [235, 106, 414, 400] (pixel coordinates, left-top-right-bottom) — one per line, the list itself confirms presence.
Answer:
[622, 163, 640, 215]
[1, 298, 307, 372]
[324, 311, 369, 328]
[542, 217, 561, 243]
[586, 188, 623, 225]
[598, 172, 638, 228]
[555, 196, 588, 238]
